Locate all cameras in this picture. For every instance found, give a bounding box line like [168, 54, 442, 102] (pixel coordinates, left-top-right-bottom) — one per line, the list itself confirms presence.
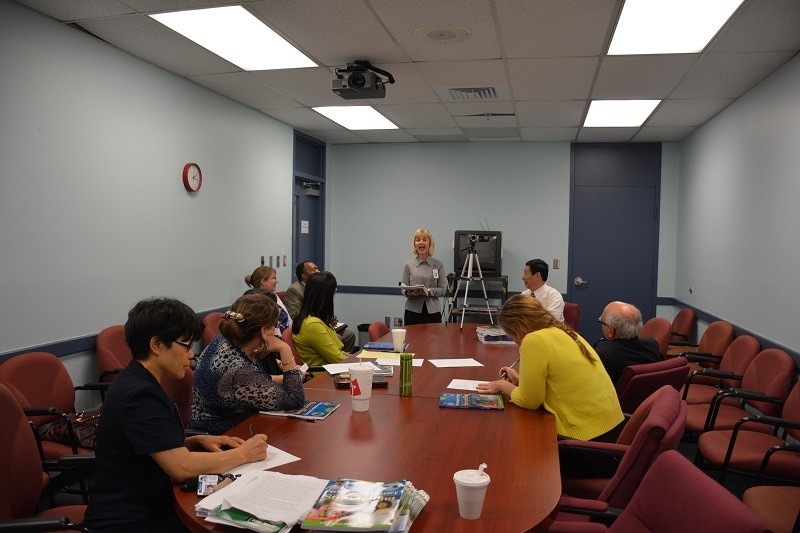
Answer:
[469, 235, 490, 244]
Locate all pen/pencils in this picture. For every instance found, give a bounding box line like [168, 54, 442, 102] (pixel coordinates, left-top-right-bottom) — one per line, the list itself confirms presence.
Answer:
[248, 424, 254, 436]
[500, 359, 520, 378]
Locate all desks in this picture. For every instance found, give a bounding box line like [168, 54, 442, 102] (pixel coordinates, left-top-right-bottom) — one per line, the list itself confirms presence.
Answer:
[172, 322, 563, 533]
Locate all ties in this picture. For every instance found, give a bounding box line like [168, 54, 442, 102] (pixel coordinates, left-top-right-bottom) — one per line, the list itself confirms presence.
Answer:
[531, 293, 535, 297]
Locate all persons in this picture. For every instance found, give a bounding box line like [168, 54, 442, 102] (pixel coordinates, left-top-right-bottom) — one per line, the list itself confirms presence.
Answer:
[245, 266, 293, 339]
[188, 291, 305, 436]
[80, 296, 268, 533]
[401, 229, 448, 326]
[291, 271, 349, 376]
[476, 294, 625, 478]
[520, 259, 565, 324]
[594, 301, 665, 387]
[207, 485, 214, 492]
[283, 260, 320, 365]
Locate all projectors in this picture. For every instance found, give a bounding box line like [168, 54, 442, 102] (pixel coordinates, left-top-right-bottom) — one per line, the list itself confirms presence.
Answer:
[331, 71, 385, 100]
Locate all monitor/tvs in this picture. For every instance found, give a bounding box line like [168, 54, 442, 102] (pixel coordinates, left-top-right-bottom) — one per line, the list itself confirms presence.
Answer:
[454, 231, 502, 277]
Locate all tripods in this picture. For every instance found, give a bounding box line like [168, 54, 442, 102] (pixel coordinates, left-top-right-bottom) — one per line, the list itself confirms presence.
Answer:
[444, 244, 494, 332]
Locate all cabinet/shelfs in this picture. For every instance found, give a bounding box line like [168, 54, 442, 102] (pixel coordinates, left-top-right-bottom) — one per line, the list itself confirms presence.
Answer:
[446, 273, 508, 322]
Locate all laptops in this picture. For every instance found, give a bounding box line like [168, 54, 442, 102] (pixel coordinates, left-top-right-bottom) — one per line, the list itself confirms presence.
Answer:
[333, 376, 387, 388]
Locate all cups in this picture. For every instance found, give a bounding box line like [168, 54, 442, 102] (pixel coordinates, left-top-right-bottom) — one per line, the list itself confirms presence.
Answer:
[392, 329, 406, 352]
[453, 469, 490, 520]
[348, 365, 374, 412]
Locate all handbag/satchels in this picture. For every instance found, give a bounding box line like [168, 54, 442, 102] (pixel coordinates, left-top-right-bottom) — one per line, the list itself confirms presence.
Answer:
[46, 410, 100, 447]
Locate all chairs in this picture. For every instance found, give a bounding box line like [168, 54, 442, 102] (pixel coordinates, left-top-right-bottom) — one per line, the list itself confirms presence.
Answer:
[547, 302, 800, 533]
[0, 292, 390, 533]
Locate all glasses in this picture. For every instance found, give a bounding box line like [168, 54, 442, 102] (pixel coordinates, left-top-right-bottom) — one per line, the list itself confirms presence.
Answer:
[175, 341, 192, 351]
[597, 317, 610, 327]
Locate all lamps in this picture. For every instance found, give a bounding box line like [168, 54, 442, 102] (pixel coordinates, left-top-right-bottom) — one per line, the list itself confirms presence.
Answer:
[182, 162, 202, 192]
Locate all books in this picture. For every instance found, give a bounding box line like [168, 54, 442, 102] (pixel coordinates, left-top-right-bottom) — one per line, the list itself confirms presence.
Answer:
[363, 342, 409, 350]
[194, 470, 430, 533]
[439, 391, 504, 410]
[259, 399, 340, 420]
[398, 281, 429, 296]
